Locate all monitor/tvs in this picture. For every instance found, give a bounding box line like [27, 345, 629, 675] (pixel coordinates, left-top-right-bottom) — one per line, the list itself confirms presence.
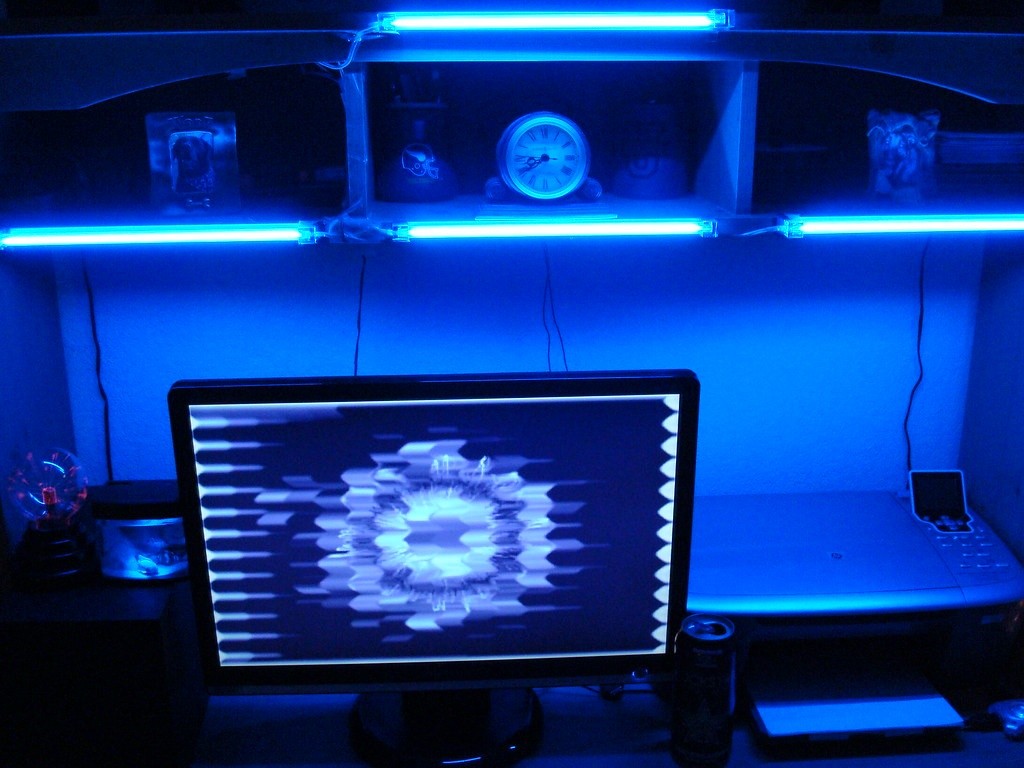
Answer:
[163, 367, 701, 768]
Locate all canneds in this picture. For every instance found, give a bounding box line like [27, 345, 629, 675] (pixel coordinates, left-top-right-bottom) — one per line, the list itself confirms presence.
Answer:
[671, 612, 737, 768]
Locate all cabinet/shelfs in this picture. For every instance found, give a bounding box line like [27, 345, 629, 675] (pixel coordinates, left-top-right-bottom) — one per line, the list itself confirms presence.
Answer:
[0, 30, 1024, 616]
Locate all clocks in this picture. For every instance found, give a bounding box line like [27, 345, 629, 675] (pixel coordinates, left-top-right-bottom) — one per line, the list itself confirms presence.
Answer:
[485, 110, 603, 202]
